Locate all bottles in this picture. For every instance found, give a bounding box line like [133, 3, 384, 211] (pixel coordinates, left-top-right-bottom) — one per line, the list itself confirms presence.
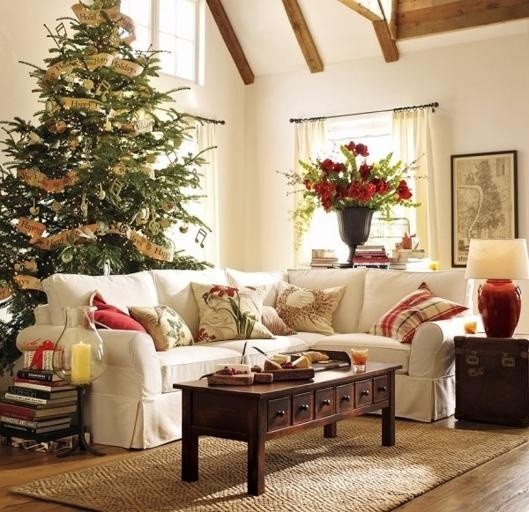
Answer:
[402, 232, 412, 249]
[51, 303, 107, 386]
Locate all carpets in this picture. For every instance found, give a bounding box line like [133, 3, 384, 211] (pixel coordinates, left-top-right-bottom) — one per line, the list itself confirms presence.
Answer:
[8, 415, 528, 512]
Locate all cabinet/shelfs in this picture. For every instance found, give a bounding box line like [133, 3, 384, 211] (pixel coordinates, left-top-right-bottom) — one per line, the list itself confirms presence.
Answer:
[453, 334, 529, 428]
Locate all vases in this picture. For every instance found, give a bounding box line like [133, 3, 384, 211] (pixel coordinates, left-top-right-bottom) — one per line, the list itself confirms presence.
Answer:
[332, 206, 377, 269]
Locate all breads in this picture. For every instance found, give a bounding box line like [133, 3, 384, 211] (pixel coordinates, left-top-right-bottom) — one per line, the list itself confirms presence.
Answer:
[215, 351, 329, 374]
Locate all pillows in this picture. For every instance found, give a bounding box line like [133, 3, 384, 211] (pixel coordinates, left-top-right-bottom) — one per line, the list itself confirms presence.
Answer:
[261, 304, 298, 335]
[124, 303, 194, 352]
[84, 290, 148, 335]
[190, 281, 275, 346]
[367, 282, 469, 343]
[274, 279, 348, 336]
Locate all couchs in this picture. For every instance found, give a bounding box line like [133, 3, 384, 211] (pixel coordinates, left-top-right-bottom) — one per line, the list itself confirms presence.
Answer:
[16, 266, 485, 450]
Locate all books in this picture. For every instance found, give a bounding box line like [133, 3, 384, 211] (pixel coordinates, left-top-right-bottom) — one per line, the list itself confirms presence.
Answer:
[310, 244, 432, 271]
[0, 370, 91, 455]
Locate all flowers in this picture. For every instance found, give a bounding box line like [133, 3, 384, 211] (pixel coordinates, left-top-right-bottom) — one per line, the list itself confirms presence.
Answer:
[275, 141, 428, 227]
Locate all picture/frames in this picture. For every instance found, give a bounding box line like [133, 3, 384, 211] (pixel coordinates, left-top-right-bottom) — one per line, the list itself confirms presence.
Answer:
[450, 150, 519, 269]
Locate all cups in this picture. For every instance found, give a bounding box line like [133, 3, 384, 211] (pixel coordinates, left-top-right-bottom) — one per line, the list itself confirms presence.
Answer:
[464, 318, 478, 336]
[351, 349, 368, 374]
[431, 262, 438, 271]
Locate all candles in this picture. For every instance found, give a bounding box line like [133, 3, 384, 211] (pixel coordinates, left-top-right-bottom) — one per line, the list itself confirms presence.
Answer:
[70, 341, 92, 385]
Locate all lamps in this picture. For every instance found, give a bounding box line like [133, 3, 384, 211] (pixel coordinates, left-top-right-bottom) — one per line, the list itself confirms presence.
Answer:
[465, 238, 529, 338]
